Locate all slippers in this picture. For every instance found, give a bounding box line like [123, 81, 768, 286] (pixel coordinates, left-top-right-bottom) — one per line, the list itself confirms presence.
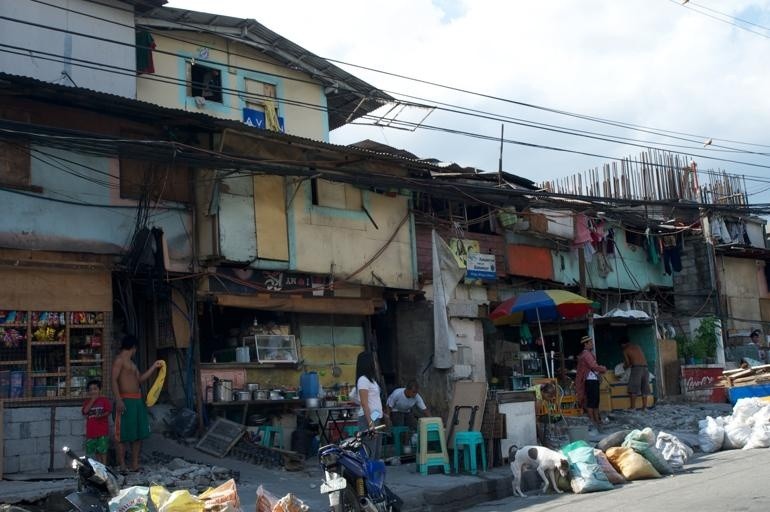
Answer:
[119, 468, 129, 475]
[130, 468, 150, 473]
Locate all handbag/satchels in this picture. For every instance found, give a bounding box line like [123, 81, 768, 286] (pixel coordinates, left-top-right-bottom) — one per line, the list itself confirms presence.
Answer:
[349, 386, 359, 404]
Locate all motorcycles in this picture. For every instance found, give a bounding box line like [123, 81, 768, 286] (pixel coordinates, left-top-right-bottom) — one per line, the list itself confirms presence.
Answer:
[316, 423, 404, 512]
[0, 446, 120, 512]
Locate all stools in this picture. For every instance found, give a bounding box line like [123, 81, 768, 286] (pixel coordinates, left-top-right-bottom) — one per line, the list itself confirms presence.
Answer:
[417, 417, 487, 475]
[255, 425, 285, 451]
[382, 426, 415, 458]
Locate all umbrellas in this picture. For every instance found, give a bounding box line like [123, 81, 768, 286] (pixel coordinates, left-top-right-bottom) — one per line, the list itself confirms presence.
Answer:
[488, 288, 594, 378]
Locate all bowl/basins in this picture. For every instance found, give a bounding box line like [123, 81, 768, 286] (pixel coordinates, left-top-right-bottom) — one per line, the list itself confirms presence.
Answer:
[304, 397, 322, 409]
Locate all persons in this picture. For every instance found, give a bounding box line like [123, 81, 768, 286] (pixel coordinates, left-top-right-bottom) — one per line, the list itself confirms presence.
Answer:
[575, 335, 607, 424]
[355, 350, 386, 462]
[618, 335, 651, 413]
[523, 382, 557, 443]
[80, 379, 113, 465]
[386, 378, 433, 446]
[750, 328, 770, 364]
[455, 238, 475, 266]
[110, 334, 164, 475]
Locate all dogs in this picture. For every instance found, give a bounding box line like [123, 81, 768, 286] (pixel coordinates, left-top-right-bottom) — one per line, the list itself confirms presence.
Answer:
[508, 445, 570, 498]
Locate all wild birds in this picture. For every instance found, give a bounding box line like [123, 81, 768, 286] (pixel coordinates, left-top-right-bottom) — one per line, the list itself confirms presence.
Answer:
[704, 137, 712, 146]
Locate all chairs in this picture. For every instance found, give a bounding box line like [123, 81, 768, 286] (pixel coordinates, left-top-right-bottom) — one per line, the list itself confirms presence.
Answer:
[528, 377, 569, 448]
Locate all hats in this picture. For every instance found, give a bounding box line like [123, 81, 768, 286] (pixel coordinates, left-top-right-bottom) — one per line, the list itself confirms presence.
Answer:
[581, 336, 592, 343]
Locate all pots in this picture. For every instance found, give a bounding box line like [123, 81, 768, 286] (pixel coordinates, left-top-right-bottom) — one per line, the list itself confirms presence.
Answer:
[213, 380, 233, 403]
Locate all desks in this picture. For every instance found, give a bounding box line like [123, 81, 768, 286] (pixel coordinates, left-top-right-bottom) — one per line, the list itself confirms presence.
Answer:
[295, 406, 361, 455]
